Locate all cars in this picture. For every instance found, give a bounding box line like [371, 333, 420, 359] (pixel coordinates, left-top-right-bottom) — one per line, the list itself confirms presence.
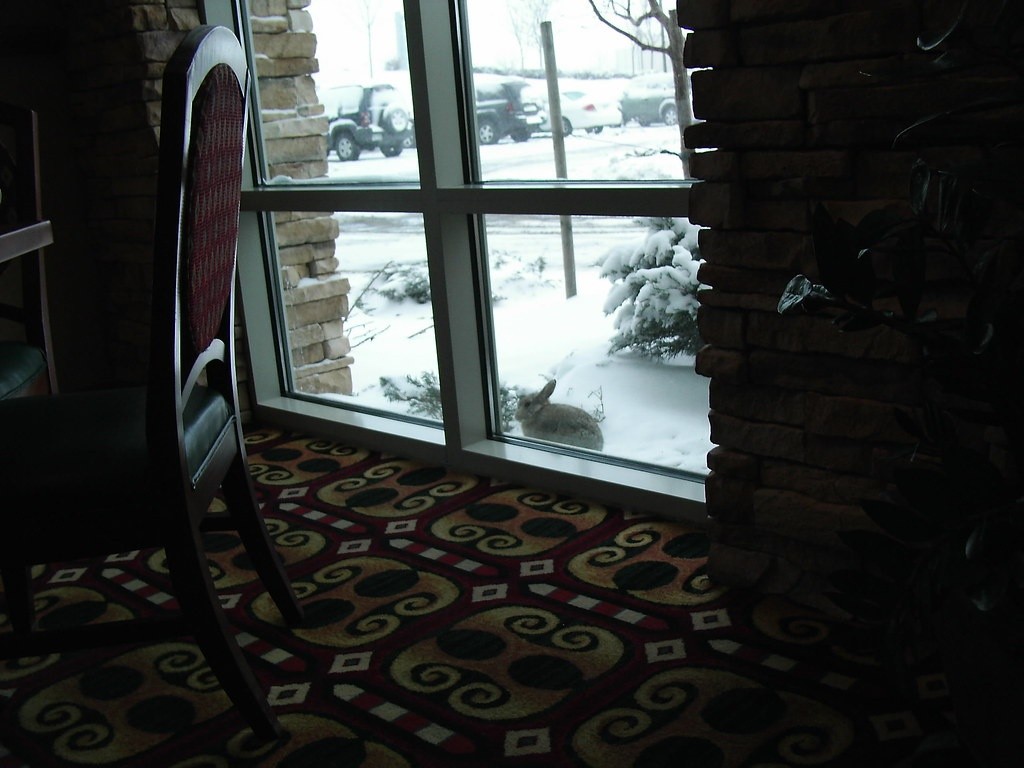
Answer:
[622, 76, 679, 126]
[542, 90, 621, 136]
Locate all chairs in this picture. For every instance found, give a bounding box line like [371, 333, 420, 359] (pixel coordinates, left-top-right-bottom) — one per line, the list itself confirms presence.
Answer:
[0, 25, 304, 737]
[0, 100, 59, 401]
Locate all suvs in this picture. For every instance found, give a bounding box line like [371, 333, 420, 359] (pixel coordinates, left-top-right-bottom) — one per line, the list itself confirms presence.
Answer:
[475, 79, 545, 143]
[326, 84, 411, 162]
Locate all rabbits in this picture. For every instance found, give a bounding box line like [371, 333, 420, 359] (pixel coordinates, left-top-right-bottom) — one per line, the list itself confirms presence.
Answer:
[513, 379, 604, 452]
